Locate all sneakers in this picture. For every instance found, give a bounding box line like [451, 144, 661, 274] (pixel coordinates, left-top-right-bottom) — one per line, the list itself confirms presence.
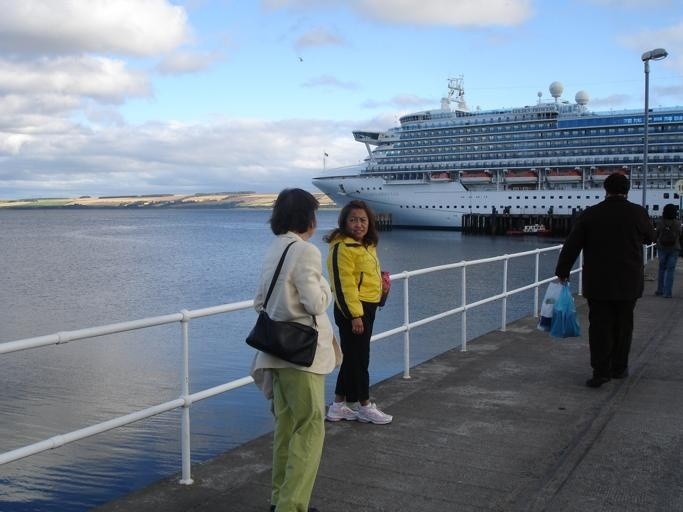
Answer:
[326, 404, 357, 421]
[586, 366, 630, 387]
[357, 403, 394, 425]
[654, 290, 673, 299]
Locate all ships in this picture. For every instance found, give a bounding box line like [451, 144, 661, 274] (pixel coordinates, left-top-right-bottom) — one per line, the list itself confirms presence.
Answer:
[308, 75, 681, 230]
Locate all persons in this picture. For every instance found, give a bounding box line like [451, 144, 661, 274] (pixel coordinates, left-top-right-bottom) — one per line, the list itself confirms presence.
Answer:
[652, 203, 683, 298]
[321, 200, 393, 425]
[553, 172, 658, 387]
[246, 186, 343, 512]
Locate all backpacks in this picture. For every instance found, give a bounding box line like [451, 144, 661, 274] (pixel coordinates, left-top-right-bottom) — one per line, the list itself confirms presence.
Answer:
[658, 218, 678, 247]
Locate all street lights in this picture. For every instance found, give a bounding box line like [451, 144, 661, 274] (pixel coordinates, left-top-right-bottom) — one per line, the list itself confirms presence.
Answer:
[639, 44, 668, 208]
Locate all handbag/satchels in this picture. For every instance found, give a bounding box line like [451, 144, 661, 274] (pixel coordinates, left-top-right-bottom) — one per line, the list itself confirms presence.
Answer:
[377, 270, 391, 308]
[245, 309, 319, 368]
[536, 280, 571, 332]
